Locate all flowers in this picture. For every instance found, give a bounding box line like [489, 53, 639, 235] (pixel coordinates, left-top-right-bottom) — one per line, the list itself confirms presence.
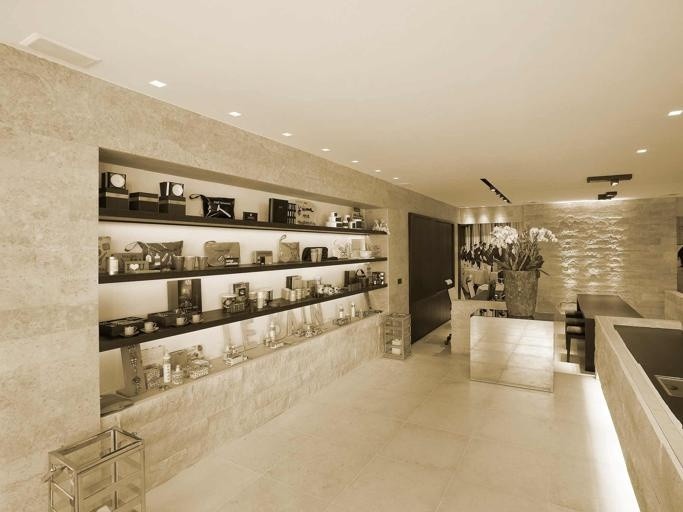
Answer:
[462, 221, 558, 278]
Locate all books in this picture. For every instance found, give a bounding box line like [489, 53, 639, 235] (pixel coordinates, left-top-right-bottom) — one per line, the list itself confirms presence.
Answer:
[268, 197, 288, 223]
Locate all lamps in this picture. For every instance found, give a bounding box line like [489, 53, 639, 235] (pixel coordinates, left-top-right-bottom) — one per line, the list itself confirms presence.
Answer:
[587, 173, 632, 199]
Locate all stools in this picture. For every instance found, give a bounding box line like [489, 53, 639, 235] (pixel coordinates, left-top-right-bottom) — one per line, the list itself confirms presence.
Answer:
[565, 311, 584, 362]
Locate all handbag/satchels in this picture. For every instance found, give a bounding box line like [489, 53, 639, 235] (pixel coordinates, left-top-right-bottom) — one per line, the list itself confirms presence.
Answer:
[203, 242, 241, 267]
[202, 196, 234, 219]
[277, 242, 301, 263]
[136, 240, 184, 270]
[302, 248, 328, 262]
[353, 276, 369, 288]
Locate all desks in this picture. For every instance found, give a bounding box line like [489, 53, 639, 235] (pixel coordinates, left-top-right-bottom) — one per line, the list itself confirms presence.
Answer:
[577, 294, 645, 373]
[451, 283, 506, 354]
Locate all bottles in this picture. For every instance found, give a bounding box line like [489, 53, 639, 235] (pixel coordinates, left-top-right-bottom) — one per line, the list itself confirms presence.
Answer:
[280, 283, 344, 301]
[162, 351, 171, 383]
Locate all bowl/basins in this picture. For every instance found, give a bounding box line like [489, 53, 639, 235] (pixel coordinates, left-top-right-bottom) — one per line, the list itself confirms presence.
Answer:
[360, 250, 373, 258]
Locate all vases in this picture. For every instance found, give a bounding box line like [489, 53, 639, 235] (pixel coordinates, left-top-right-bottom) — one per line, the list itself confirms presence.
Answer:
[499, 269, 539, 316]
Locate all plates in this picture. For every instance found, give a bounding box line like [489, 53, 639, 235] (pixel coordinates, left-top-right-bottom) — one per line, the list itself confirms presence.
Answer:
[121, 331, 139, 337]
[173, 322, 189, 326]
[359, 256, 375, 259]
[141, 327, 159, 333]
[189, 319, 205, 324]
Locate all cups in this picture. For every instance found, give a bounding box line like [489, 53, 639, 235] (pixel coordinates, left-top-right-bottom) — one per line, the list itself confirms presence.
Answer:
[142, 321, 157, 329]
[174, 254, 208, 271]
[124, 326, 136, 334]
[311, 246, 322, 263]
[192, 314, 203, 322]
[175, 317, 186, 324]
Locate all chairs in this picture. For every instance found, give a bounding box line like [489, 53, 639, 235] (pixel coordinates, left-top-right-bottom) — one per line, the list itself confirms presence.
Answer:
[444, 271, 499, 345]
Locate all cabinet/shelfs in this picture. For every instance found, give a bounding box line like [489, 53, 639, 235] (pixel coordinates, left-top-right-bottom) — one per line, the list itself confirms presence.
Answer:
[99, 208, 387, 416]
[40, 427, 147, 511]
[384, 314, 411, 361]
